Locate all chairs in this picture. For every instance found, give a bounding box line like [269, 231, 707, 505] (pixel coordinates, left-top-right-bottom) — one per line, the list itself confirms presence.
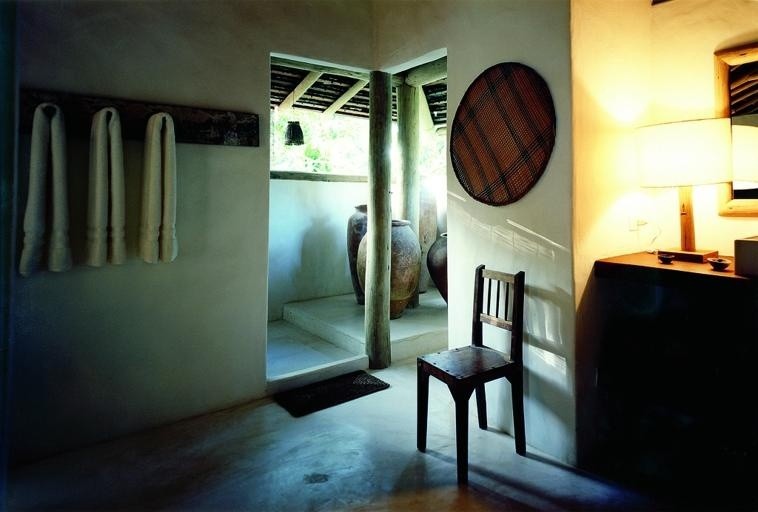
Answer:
[416, 264, 526, 487]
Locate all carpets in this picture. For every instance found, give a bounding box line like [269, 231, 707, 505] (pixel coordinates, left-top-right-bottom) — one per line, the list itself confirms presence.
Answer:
[272, 370, 390, 418]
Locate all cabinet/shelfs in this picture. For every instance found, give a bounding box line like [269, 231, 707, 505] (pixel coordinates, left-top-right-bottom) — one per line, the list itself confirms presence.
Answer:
[594, 246, 758, 512]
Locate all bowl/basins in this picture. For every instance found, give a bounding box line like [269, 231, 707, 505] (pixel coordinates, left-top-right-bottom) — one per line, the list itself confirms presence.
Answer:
[658, 254, 676, 265]
[707, 257, 732, 270]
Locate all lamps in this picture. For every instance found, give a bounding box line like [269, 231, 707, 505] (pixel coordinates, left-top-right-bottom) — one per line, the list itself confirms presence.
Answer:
[283, 86, 304, 146]
[633, 114, 733, 265]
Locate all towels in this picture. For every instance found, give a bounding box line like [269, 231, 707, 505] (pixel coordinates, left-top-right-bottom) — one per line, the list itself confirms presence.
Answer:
[19, 102, 178, 278]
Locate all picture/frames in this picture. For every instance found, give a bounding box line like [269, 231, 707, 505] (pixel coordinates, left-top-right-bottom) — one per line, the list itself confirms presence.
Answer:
[713, 46, 758, 216]
